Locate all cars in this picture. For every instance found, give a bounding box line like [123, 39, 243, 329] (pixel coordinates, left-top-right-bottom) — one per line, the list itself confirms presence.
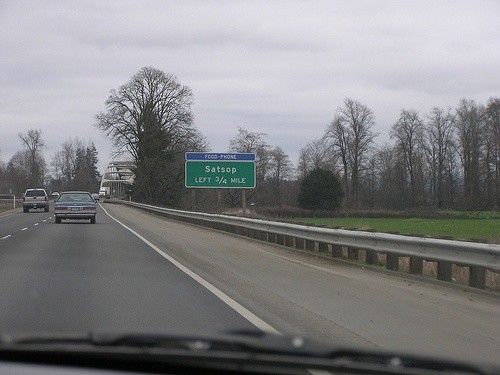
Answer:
[53, 191, 98, 224]
[92, 193, 100, 201]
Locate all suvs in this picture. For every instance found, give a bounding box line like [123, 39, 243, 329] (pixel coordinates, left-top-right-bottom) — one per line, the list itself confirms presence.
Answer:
[23, 189, 50, 213]
